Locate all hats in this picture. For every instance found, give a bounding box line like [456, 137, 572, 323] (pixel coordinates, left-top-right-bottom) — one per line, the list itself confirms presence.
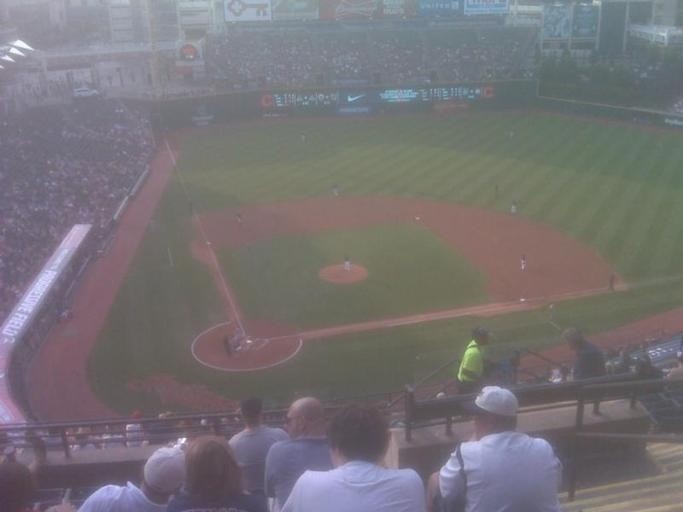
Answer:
[143, 447, 186, 495]
[474, 385, 518, 417]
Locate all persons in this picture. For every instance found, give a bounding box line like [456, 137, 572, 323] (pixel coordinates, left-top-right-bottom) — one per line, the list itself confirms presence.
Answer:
[521, 254, 528, 270]
[608, 275, 615, 291]
[331, 183, 340, 197]
[563, 325, 682, 427]
[344, 253, 349, 272]
[0, 26, 159, 325]
[511, 200, 519, 214]
[161, 89, 210, 97]
[237, 211, 242, 223]
[3, 325, 562, 511]
[214, 27, 539, 92]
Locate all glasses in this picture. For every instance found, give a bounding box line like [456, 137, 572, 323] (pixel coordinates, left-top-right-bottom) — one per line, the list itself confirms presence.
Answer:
[282, 414, 295, 424]
